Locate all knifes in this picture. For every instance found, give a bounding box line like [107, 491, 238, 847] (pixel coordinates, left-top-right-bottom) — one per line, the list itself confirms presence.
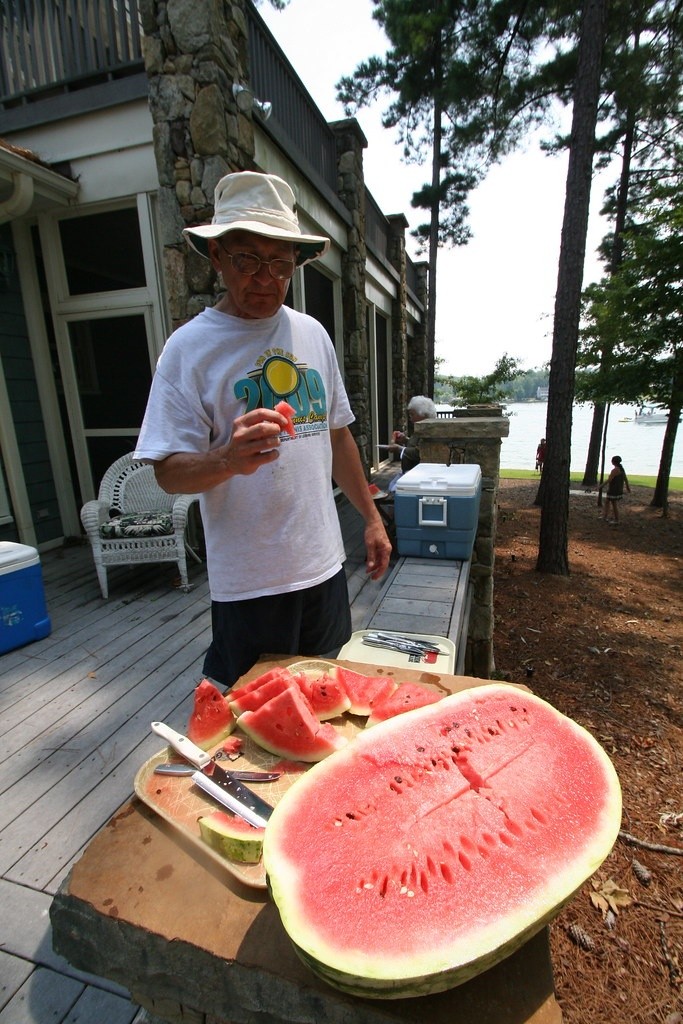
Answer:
[154, 763, 281, 782]
[151, 721, 274, 828]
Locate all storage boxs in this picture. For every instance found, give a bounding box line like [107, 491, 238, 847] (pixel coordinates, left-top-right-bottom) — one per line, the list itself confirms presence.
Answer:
[0, 540, 51, 656]
[396, 463, 482, 559]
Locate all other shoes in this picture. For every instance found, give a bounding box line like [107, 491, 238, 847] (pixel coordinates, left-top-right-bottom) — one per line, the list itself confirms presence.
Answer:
[598, 517, 608, 522]
[609, 520, 619, 524]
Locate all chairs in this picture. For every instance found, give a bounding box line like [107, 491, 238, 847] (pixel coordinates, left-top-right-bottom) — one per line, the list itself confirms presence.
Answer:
[80, 451, 199, 598]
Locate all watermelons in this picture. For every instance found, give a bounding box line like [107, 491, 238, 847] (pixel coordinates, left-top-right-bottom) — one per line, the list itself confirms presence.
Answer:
[274, 400, 297, 438]
[185, 665, 623, 999]
[368, 484, 382, 496]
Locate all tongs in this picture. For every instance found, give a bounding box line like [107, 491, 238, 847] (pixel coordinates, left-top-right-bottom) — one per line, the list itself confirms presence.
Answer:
[362, 632, 450, 656]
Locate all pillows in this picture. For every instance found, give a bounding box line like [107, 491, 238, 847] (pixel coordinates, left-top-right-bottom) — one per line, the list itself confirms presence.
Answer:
[98, 511, 174, 536]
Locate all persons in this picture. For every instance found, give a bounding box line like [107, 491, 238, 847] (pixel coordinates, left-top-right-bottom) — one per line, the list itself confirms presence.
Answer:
[132, 170, 392, 685]
[536, 439, 546, 469]
[386, 395, 437, 474]
[595, 456, 631, 525]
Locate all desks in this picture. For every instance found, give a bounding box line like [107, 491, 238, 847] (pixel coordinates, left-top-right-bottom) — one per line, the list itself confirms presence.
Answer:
[48, 652, 564, 1024]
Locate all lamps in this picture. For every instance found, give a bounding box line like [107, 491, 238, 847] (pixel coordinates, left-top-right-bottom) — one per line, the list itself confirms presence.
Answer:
[233, 81, 273, 122]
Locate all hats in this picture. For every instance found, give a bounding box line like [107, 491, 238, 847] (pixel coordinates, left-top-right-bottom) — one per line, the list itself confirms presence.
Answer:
[182, 170, 330, 270]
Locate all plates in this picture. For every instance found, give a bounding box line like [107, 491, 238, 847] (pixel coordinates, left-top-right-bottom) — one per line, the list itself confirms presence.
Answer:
[372, 493, 388, 499]
[376, 445, 389, 448]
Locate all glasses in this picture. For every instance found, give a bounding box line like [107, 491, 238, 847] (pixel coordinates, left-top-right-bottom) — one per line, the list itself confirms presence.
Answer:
[218, 239, 300, 280]
